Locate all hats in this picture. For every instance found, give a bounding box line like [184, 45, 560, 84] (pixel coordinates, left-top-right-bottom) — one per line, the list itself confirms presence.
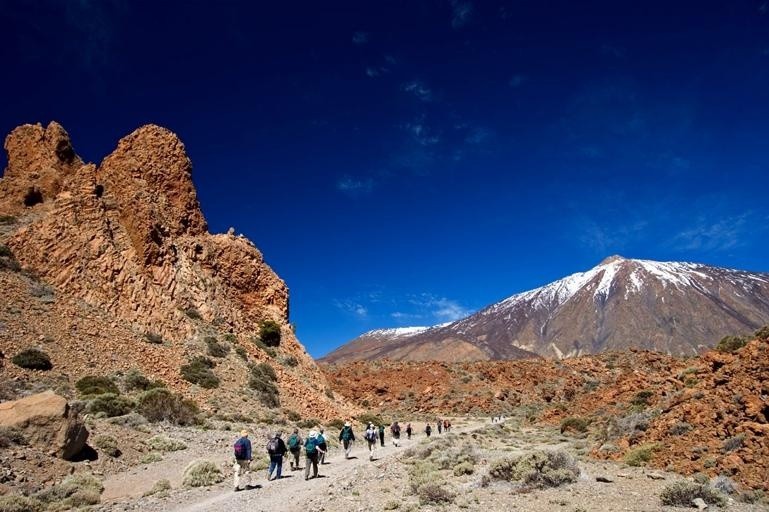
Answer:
[309, 431, 318, 438]
[344, 422, 351, 427]
[241, 429, 249, 438]
[234, 463, 326, 492]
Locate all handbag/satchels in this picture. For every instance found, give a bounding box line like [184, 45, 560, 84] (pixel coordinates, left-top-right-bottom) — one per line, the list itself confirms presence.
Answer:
[233, 439, 249, 461]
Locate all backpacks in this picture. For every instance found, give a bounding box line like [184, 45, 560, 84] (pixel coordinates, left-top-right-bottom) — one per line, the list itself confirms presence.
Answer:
[306, 439, 316, 455]
[343, 428, 351, 440]
[317, 434, 325, 445]
[269, 438, 281, 454]
[366, 429, 377, 442]
[289, 435, 298, 451]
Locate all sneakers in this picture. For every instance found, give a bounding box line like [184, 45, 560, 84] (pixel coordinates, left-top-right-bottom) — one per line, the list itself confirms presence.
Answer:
[345, 437, 412, 462]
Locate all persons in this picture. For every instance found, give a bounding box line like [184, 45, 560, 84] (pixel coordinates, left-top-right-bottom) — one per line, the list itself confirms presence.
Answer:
[379, 423, 386, 447]
[406, 423, 414, 439]
[338, 421, 355, 459]
[303, 431, 325, 479]
[366, 421, 373, 430]
[436, 420, 442, 433]
[286, 429, 304, 469]
[390, 421, 401, 447]
[424, 422, 431, 436]
[365, 423, 378, 460]
[265, 430, 288, 479]
[316, 429, 328, 464]
[232, 429, 253, 490]
